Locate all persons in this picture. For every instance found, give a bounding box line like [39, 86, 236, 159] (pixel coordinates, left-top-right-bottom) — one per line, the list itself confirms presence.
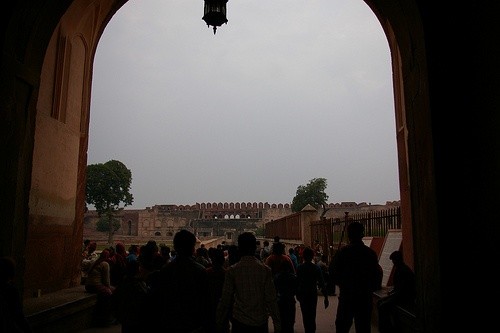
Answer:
[0, 206, 500, 332]
